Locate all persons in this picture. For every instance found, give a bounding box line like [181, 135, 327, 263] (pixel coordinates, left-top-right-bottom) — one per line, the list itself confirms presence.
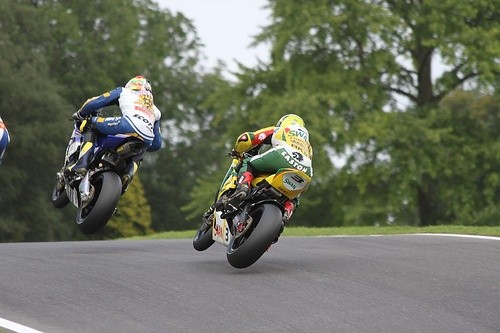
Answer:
[226, 114, 313, 238]
[0, 116, 11, 159]
[63, 76, 161, 195]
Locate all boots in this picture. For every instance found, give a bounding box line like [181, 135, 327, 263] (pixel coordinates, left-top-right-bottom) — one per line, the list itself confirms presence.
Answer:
[66, 141, 93, 174]
[272, 201, 295, 244]
[226, 171, 252, 210]
[119, 162, 138, 196]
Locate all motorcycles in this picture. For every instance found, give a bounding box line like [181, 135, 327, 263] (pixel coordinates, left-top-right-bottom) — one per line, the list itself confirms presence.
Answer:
[193, 132, 312, 268]
[52, 112, 139, 234]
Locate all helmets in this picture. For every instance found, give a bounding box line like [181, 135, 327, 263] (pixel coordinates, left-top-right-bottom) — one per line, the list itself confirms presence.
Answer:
[125, 75, 151, 92]
[277, 113, 305, 128]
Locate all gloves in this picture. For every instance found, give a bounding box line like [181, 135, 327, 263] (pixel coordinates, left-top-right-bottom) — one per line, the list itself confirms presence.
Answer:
[231, 149, 244, 159]
[72, 111, 87, 121]
